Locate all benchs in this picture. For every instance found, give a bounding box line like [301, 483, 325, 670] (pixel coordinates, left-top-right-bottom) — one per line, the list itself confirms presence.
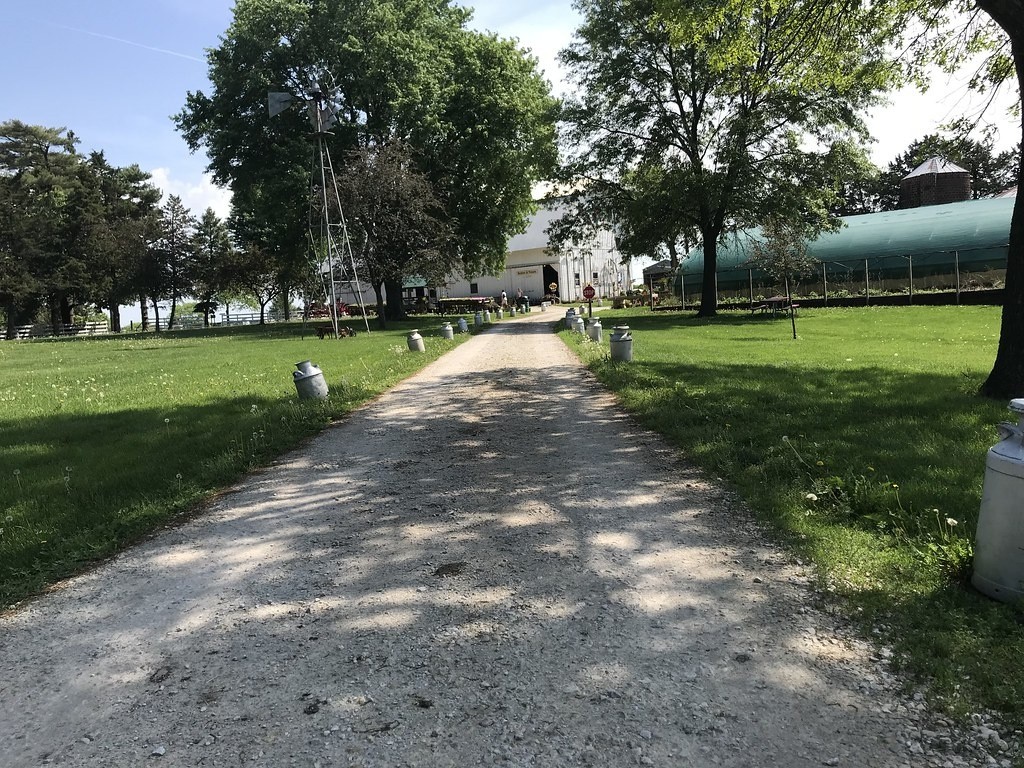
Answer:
[783, 304, 798, 317]
[750, 304, 769, 317]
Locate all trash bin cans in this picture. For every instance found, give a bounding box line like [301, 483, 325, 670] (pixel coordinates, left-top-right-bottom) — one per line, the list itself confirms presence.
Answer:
[521, 295, 531, 312]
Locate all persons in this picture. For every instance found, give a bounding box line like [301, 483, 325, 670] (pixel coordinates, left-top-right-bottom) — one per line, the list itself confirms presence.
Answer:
[517, 288, 523, 299]
[501, 289, 509, 307]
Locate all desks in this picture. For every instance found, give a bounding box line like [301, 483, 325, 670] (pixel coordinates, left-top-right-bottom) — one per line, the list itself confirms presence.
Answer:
[759, 297, 793, 316]
